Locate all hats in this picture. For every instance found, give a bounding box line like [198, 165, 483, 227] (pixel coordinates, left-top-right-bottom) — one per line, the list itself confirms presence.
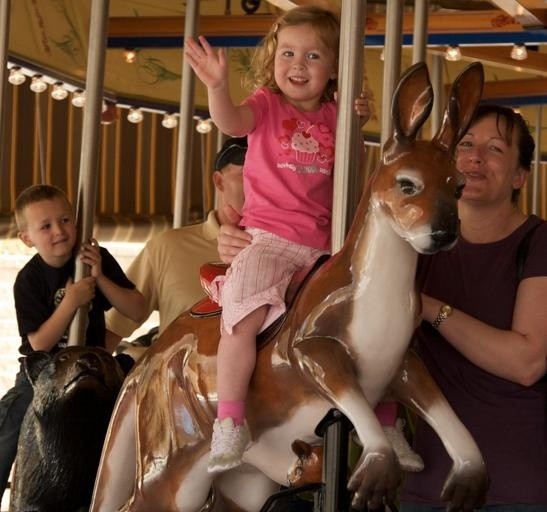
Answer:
[215, 138, 247, 170]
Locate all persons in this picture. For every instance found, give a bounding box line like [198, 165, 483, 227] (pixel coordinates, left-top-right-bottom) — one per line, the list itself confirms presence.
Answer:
[389, 102, 547, 510]
[0, 185, 146, 500]
[184, 5, 427, 475]
[104, 137, 247, 354]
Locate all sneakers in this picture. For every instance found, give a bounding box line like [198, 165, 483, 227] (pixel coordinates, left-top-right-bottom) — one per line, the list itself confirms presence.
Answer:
[205, 417, 253, 473]
[353, 417, 425, 472]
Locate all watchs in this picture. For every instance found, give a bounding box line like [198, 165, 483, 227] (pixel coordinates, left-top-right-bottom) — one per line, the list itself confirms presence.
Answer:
[432, 304, 451, 328]
